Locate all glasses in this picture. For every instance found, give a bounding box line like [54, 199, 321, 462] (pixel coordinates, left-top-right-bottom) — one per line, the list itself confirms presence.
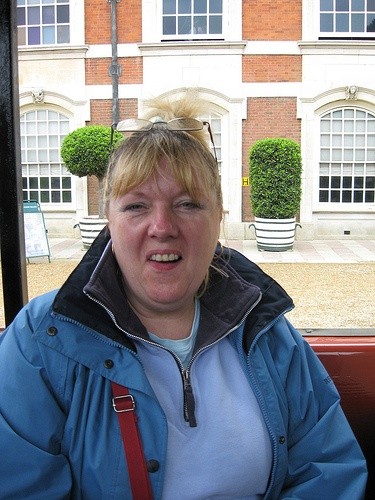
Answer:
[110, 117, 217, 161]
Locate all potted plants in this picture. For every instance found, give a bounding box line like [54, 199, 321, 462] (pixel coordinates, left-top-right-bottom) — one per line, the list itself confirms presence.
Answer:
[249, 137, 304, 253]
[61, 125, 125, 252]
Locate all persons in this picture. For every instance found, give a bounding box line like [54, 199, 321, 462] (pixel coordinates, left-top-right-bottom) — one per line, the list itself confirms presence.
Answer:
[1, 97, 369, 500]
